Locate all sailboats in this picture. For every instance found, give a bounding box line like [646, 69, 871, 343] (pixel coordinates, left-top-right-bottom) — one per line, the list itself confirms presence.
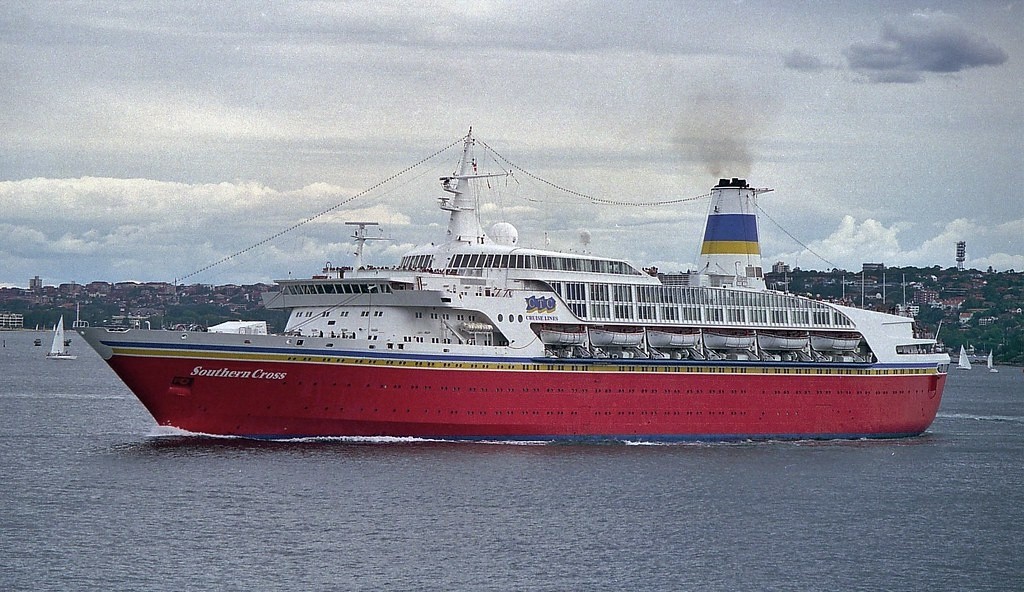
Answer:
[955, 344, 972, 370]
[986, 349, 999, 373]
[45, 313, 78, 360]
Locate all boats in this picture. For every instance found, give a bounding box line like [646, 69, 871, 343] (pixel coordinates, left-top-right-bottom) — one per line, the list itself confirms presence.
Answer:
[71, 126, 952, 443]
